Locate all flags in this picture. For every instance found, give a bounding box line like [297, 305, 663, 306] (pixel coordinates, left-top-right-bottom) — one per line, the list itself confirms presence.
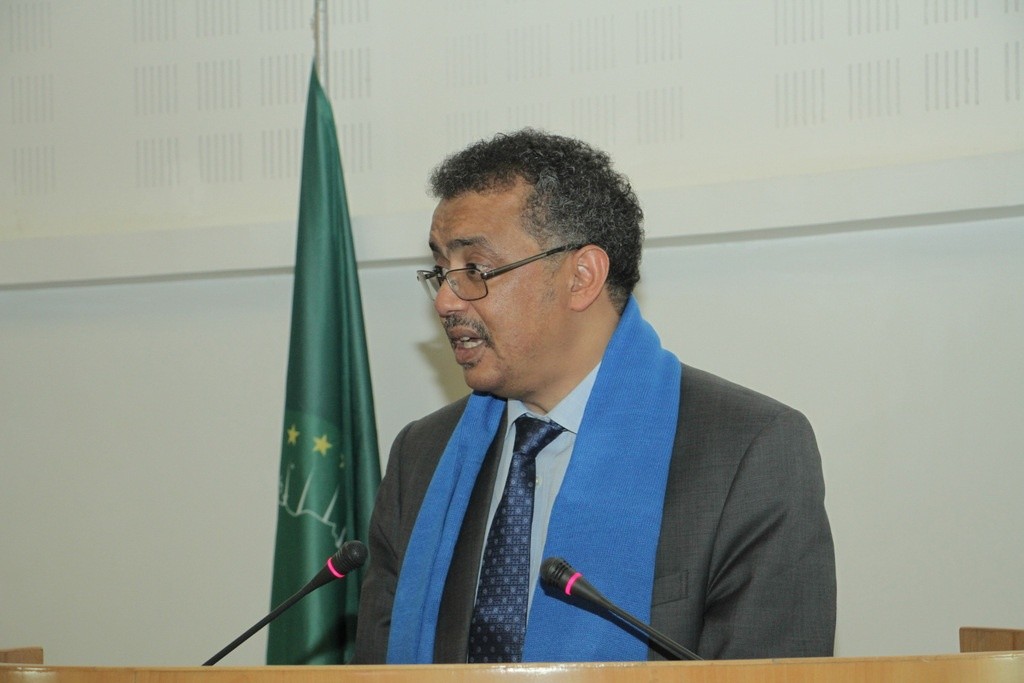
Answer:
[265, 64, 382, 664]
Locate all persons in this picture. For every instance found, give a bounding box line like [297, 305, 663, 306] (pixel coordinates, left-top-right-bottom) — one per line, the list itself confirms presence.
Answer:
[354, 135, 839, 666]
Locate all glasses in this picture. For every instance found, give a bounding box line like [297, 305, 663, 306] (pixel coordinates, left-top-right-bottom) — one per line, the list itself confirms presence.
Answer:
[415, 240, 605, 301]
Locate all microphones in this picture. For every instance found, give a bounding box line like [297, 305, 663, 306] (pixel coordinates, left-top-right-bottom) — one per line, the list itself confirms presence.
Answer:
[541, 558, 705, 661]
[201, 541, 368, 666]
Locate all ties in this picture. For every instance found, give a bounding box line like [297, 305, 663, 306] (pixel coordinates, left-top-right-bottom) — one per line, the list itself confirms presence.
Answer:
[464, 412, 569, 663]
